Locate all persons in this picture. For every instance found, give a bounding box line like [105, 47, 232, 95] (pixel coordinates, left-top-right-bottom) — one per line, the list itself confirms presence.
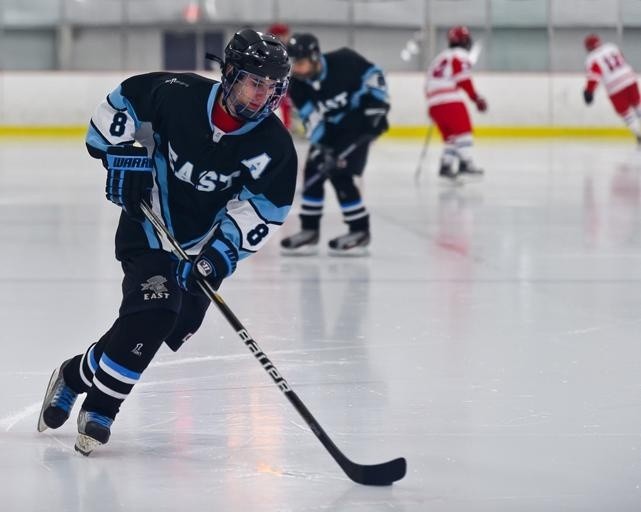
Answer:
[424, 23, 491, 178]
[265, 20, 294, 131]
[44, 27, 299, 446]
[580, 33, 641, 147]
[281, 32, 392, 249]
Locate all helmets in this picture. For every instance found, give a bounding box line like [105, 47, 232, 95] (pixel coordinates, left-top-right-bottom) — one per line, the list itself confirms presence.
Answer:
[287, 33, 321, 80]
[585, 35, 603, 50]
[205, 29, 291, 105]
[449, 26, 470, 45]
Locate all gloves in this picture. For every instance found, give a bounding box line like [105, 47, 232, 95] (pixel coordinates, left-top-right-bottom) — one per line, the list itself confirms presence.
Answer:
[584, 91, 592, 103]
[320, 144, 354, 177]
[105, 147, 153, 222]
[176, 237, 239, 298]
[477, 100, 489, 111]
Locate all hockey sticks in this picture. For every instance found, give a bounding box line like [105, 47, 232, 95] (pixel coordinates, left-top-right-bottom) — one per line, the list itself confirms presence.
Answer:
[141, 196, 406, 484]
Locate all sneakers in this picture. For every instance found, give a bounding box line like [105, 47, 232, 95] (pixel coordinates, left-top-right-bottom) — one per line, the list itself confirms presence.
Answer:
[459, 160, 483, 174]
[440, 157, 457, 177]
[43, 355, 78, 429]
[77, 409, 113, 443]
[280, 232, 319, 249]
[329, 232, 370, 249]
